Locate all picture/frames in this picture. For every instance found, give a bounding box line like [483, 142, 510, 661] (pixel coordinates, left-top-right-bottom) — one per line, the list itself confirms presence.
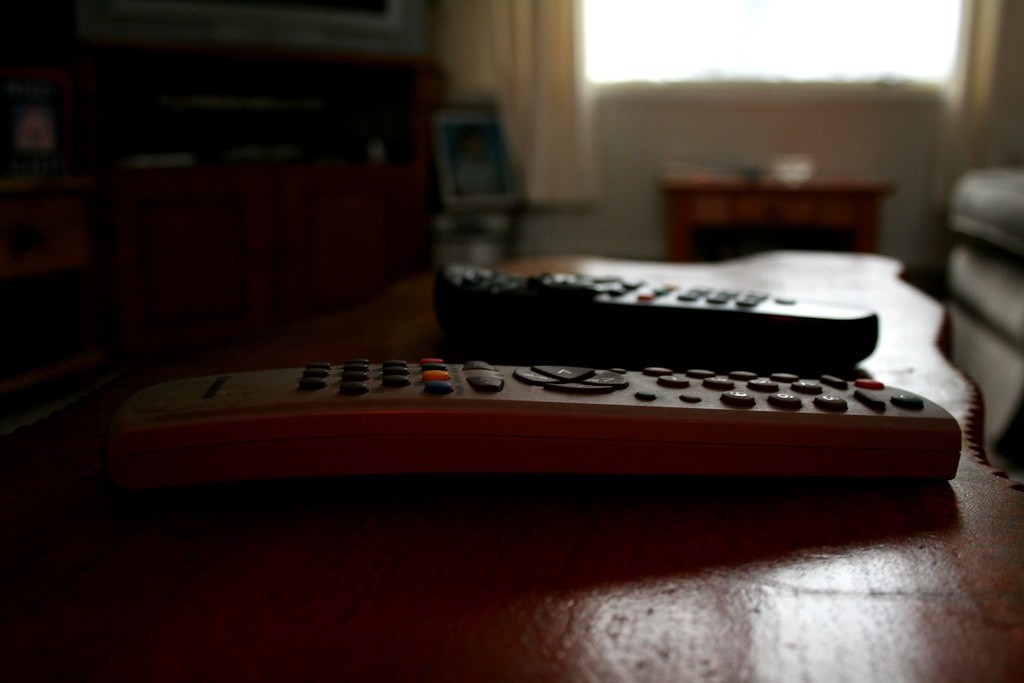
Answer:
[426, 93, 532, 203]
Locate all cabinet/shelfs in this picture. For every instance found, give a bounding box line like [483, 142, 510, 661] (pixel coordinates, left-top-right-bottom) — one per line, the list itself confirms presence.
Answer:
[69, 34, 432, 361]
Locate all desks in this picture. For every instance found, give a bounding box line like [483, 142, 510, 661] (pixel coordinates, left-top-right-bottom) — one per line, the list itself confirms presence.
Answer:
[436, 196, 597, 271]
[657, 174, 891, 263]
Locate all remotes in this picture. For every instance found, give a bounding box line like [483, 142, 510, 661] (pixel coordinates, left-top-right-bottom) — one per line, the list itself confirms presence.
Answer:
[102, 359, 961, 490]
[434, 265, 878, 373]
